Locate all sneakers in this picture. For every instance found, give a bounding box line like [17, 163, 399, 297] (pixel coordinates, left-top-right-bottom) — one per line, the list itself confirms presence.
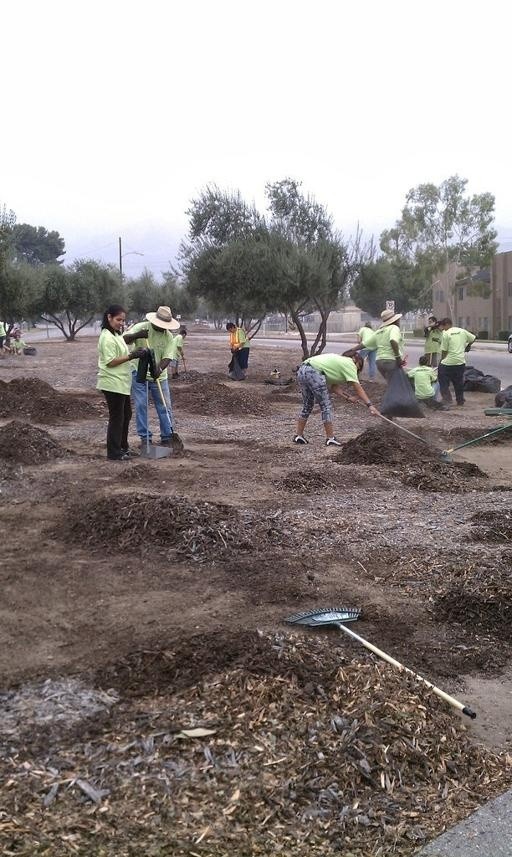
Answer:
[441, 399, 463, 406]
[326, 436, 342, 446]
[293, 434, 308, 444]
[141, 440, 152, 448]
[161, 440, 169, 447]
[109, 449, 138, 459]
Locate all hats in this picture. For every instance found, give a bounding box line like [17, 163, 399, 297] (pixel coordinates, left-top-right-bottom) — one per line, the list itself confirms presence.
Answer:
[146, 306, 180, 329]
[380, 310, 402, 329]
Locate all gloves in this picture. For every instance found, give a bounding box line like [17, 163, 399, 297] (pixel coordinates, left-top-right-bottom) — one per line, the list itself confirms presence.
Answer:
[129, 350, 145, 360]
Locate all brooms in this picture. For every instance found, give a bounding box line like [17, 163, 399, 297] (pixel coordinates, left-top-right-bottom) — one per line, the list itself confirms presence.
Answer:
[142, 327, 184, 450]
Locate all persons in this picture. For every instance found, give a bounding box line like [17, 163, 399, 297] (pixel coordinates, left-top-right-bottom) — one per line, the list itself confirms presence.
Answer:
[95, 305, 187, 460]
[0, 321, 29, 356]
[226, 323, 250, 378]
[293, 310, 476, 446]
